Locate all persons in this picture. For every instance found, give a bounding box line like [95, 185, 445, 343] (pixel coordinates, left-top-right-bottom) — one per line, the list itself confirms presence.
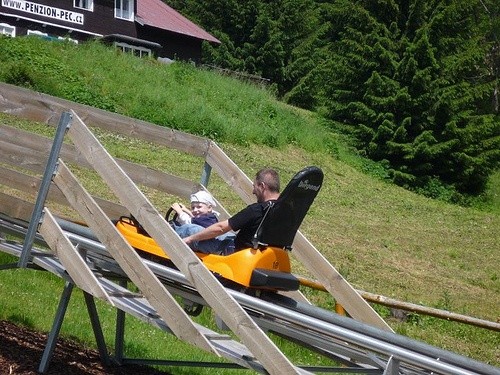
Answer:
[174, 168, 282, 256]
[170, 190, 219, 228]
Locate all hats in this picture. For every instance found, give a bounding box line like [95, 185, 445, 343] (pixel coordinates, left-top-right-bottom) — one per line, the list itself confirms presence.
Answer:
[189, 192, 218, 209]
